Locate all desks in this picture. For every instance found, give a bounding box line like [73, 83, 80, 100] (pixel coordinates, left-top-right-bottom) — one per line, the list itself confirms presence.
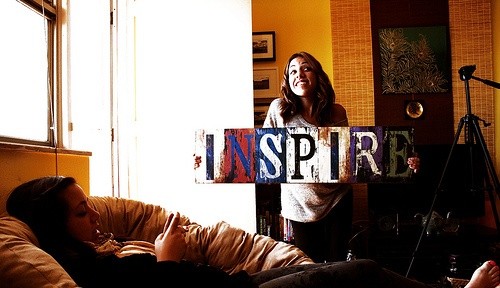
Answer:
[351, 182, 412, 260]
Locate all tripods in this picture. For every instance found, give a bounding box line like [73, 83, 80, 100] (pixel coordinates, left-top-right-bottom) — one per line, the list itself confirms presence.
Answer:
[406, 64, 500, 279]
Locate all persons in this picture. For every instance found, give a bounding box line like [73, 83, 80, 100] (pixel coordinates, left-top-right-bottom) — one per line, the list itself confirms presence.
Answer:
[261, 51, 351, 263]
[7, 177, 494, 288]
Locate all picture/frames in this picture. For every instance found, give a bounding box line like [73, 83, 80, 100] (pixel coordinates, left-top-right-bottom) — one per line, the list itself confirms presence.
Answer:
[255, 103, 271, 125]
[253, 66, 281, 99]
[252, 31, 276, 62]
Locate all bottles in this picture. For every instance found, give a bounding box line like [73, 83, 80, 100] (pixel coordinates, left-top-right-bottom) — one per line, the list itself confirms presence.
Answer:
[447, 254, 461, 279]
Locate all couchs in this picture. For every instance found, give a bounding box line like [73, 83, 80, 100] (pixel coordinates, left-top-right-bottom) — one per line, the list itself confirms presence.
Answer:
[0, 196, 317, 287]
